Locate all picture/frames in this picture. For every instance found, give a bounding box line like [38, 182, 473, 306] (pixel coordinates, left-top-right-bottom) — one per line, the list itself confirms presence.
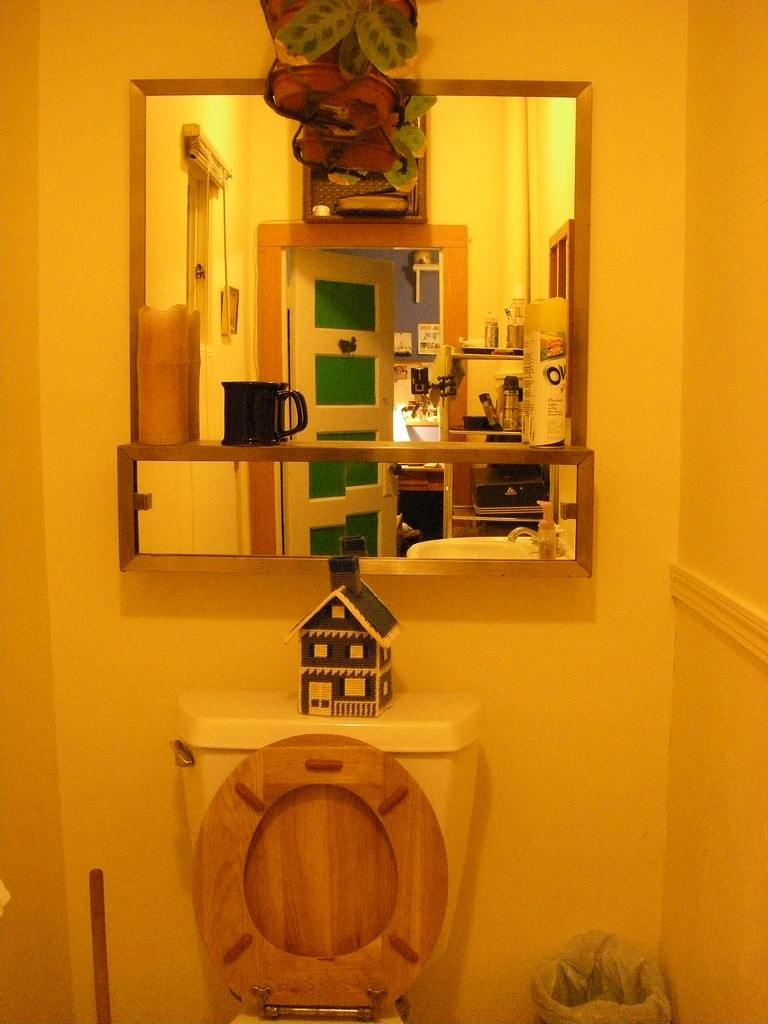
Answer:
[220, 286, 240, 334]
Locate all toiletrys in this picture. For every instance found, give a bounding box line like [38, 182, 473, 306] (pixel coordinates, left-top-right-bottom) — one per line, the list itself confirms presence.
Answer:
[536, 500, 557, 559]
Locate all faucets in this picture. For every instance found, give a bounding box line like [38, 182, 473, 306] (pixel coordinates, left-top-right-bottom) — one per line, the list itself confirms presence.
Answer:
[508, 527, 538, 543]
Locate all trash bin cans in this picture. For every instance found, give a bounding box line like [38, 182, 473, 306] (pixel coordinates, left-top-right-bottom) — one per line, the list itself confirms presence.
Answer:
[530, 928, 672, 1024]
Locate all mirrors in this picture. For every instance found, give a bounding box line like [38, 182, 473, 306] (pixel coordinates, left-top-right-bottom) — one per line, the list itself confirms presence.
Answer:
[119, 78, 600, 576]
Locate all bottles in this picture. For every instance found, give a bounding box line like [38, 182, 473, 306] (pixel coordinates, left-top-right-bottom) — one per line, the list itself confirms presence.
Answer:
[523, 304, 530, 444]
[502, 375, 519, 431]
[484, 322, 498, 348]
[528, 297, 569, 450]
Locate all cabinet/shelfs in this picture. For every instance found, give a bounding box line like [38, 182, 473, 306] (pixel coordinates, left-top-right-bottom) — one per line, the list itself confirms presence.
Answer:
[443, 344, 541, 543]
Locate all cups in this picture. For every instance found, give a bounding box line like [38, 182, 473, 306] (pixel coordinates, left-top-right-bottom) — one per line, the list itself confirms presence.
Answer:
[278, 383, 308, 442]
[220, 381, 303, 446]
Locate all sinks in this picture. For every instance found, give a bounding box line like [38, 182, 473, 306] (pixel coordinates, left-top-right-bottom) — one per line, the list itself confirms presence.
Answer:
[405, 535, 539, 559]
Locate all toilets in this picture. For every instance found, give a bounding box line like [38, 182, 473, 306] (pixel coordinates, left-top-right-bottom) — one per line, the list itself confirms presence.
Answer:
[167, 683, 487, 1024]
